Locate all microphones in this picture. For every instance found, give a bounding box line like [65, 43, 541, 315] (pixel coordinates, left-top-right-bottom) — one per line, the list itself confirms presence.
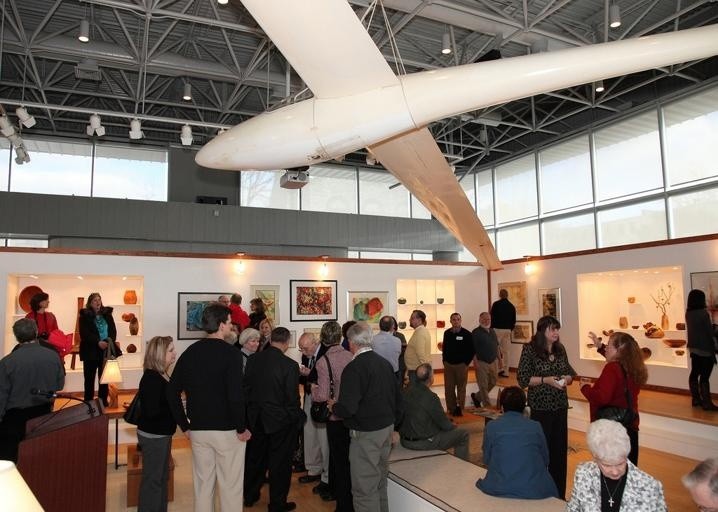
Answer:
[31, 388, 48, 396]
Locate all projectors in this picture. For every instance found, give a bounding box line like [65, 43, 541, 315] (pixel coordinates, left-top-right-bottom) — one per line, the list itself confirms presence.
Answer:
[280, 171, 309, 189]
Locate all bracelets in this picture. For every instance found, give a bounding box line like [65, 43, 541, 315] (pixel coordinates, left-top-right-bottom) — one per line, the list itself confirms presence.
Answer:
[541, 376, 544, 384]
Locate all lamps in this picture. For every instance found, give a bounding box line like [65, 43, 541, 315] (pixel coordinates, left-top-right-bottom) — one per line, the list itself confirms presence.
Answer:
[13, 108, 36, 130]
[595, 81, 606, 92]
[607, 4, 624, 28]
[130, 122, 142, 141]
[0, 115, 17, 138]
[180, 125, 194, 146]
[181, 84, 195, 101]
[99, 360, 123, 408]
[86, 114, 107, 137]
[14, 139, 30, 164]
[78, 21, 94, 42]
[439, 35, 454, 55]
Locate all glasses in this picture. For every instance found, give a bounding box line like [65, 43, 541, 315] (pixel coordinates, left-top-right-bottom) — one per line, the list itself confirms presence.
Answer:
[298, 345, 313, 353]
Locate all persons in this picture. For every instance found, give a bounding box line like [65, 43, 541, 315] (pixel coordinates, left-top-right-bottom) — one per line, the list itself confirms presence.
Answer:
[680, 457, 718, 512]
[516, 316, 577, 502]
[476, 386, 559, 500]
[137, 287, 517, 512]
[78, 292, 116, 406]
[579, 331, 648, 466]
[562, 420, 668, 512]
[685, 290, 718, 411]
[0, 318, 64, 464]
[25, 292, 58, 340]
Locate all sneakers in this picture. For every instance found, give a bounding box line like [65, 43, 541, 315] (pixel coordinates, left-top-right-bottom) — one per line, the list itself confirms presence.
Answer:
[501, 375, 509, 378]
[498, 370, 505, 376]
[299, 474, 321, 484]
[312, 481, 337, 501]
[702, 402, 718, 411]
[471, 393, 480, 408]
[244, 492, 261, 507]
[692, 398, 704, 407]
[268, 502, 296, 512]
[98, 398, 109, 407]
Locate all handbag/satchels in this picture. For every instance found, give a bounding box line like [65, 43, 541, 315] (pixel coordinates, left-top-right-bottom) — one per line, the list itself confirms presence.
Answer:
[105, 342, 123, 360]
[596, 404, 636, 430]
[310, 400, 333, 424]
[122, 390, 143, 426]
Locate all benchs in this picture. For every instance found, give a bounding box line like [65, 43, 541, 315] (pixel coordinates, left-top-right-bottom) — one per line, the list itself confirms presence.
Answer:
[388, 446, 568, 512]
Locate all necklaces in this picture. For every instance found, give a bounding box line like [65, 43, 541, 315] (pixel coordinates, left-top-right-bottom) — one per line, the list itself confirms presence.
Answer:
[602, 477, 622, 507]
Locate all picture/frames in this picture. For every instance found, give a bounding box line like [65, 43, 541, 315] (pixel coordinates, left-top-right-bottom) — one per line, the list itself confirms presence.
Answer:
[495, 281, 530, 316]
[176, 291, 237, 341]
[346, 291, 389, 322]
[288, 329, 298, 348]
[508, 320, 535, 345]
[303, 327, 322, 340]
[247, 284, 280, 326]
[689, 270, 718, 325]
[289, 278, 338, 323]
[537, 287, 563, 326]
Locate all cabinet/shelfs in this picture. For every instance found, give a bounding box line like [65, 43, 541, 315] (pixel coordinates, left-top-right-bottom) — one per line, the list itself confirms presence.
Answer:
[394, 279, 456, 356]
[604, 328, 688, 368]
[109, 304, 142, 355]
